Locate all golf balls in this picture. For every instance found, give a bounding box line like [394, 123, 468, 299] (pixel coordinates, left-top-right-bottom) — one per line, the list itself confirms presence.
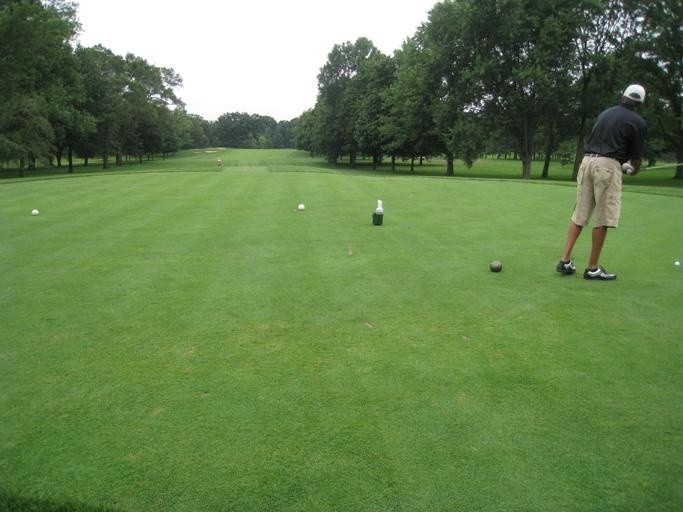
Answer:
[675, 260, 682, 267]
[298, 204, 305, 209]
[31, 209, 39, 216]
[490, 260, 503, 272]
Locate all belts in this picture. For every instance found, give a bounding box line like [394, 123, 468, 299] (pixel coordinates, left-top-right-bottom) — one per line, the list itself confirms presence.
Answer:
[585, 152, 606, 158]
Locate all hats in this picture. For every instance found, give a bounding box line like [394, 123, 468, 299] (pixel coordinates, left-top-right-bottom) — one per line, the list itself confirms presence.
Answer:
[624, 84, 646, 104]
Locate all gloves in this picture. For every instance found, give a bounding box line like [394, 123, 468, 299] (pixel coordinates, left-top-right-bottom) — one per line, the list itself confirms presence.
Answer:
[622, 163, 633, 175]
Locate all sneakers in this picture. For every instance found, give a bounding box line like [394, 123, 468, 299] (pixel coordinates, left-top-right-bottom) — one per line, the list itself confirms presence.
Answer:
[583, 264, 617, 281]
[556, 260, 577, 274]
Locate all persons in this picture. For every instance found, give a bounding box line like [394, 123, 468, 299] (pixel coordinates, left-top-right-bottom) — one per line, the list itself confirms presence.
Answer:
[553, 82, 650, 282]
